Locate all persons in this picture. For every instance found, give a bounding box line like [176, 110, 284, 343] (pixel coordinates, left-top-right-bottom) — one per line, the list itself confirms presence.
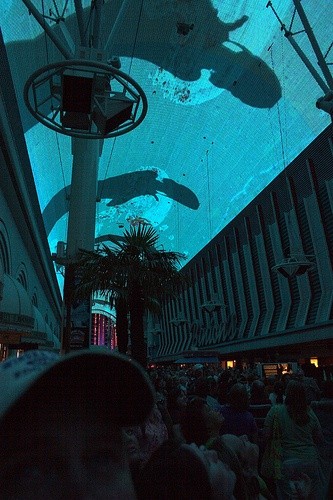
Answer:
[0, 350, 333, 500]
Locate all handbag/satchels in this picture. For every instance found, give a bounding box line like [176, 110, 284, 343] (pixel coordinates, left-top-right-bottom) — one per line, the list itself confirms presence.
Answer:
[261, 404, 283, 480]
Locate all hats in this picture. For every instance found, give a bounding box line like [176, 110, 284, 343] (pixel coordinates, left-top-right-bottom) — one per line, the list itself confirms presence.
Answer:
[0, 351, 155, 430]
[193, 364, 203, 371]
